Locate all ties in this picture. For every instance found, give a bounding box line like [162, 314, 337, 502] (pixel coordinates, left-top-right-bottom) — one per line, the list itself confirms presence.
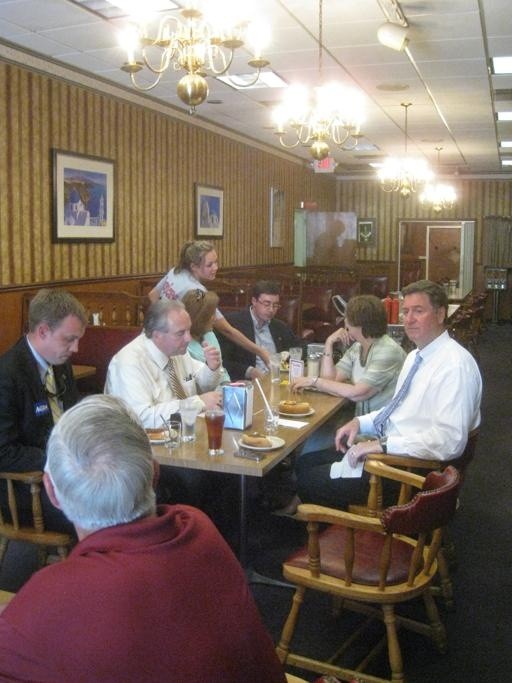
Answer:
[169, 358, 188, 400]
[373, 351, 424, 440]
[43, 365, 62, 427]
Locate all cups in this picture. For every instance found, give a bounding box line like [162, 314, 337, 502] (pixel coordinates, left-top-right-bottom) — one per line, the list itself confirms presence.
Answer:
[263, 402, 279, 432]
[269, 353, 282, 382]
[180, 408, 198, 443]
[163, 420, 181, 448]
[289, 347, 302, 360]
[307, 354, 321, 378]
[205, 411, 226, 454]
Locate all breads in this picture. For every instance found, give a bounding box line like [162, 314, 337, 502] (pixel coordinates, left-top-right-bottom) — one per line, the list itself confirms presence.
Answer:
[145, 429, 166, 440]
[243, 433, 272, 447]
[278, 401, 308, 413]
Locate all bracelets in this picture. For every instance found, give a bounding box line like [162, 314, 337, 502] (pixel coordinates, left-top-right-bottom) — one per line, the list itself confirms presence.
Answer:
[309, 375, 319, 390]
[320, 352, 335, 359]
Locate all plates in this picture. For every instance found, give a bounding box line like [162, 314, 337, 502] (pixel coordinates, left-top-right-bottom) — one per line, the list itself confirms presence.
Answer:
[142, 429, 177, 444]
[239, 434, 284, 450]
[274, 406, 315, 418]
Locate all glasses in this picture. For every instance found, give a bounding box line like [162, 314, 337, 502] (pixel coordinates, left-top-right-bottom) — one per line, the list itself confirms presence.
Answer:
[196, 288, 205, 300]
[257, 298, 282, 310]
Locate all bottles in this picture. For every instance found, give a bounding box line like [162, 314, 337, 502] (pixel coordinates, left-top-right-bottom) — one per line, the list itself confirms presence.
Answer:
[383, 295, 392, 323]
[391, 296, 399, 324]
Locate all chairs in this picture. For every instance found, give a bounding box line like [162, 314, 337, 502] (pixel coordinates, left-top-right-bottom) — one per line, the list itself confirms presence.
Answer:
[0, 469, 72, 608]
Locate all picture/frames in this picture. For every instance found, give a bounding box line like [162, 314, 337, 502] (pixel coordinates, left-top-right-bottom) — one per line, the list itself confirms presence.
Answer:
[50, 146, 115, 243]
[193, 182, 225, 239]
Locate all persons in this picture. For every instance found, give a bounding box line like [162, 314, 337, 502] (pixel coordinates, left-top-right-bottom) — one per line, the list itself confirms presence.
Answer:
[0, 392, 288, 682]
[292, 275, 484, 528]
[0, 287, 89, 548]
[312, 218, 345, 263]
[174, 288, 235, 392]
[214, 278, 309, 384]
[147, 238, 278, 369]
[270, 294, 406, 521]
[104, 298, 226, 432]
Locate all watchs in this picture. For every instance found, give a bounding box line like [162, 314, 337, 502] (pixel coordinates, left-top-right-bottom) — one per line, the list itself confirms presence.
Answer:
[379, 437, 389, 453]
